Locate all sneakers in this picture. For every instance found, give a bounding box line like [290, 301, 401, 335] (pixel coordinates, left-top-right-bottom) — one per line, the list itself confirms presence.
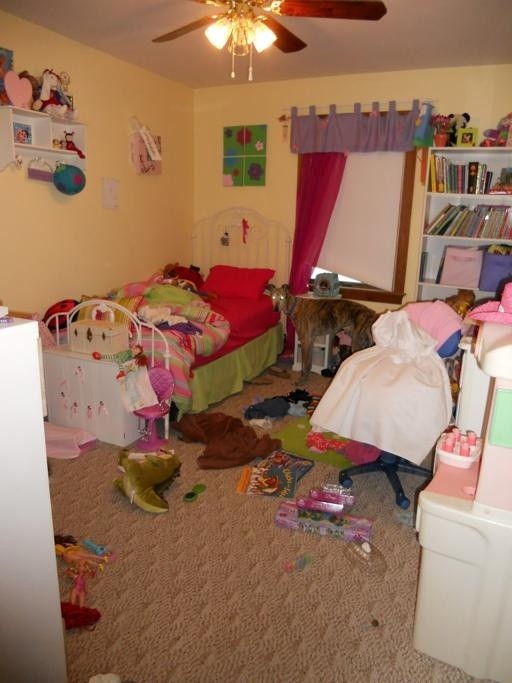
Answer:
[269, 366, 290, 377]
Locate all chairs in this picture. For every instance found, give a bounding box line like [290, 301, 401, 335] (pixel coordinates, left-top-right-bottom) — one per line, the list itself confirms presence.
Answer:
[339, 301, 460, 510]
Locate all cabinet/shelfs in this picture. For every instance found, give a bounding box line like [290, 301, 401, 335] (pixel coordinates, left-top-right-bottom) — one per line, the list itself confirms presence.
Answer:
[292, 291, 342, 377]
[1, 104, 89, 175]
[414, 146, 512, 318]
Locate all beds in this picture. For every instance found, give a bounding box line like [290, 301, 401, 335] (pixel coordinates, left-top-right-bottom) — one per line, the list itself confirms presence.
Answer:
[40, 207, 291, 441]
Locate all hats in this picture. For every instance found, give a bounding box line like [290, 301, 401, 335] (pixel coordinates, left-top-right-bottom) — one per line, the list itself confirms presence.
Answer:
[468, 282, 512, 323]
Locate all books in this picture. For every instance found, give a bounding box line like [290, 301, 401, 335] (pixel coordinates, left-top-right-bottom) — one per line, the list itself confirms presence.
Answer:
[235, 466, 297, 500]
[424, 154, 512, 242]
[247, 450, 315, 482]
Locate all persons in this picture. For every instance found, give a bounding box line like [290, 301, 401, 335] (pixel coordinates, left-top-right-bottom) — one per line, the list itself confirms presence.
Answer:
[54, 533, 117, 608]
[92, 346, 148, 380]
[53, 130, 85, 160]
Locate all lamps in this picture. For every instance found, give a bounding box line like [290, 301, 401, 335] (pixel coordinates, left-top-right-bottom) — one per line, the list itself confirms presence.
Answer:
[205, 2, 282, 54]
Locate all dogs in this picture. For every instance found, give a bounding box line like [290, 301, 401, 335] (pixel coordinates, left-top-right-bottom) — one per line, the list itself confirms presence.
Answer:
[263, 284, 379, 386]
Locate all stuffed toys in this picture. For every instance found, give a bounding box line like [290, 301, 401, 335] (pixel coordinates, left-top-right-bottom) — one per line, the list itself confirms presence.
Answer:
[112, 447, 183, 513]
[446, 112, 472, 147]
[479, 112, 511, 147]
[0, 68, 77, 120]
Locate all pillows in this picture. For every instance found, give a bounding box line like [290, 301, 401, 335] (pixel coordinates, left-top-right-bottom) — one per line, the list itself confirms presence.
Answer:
[76, 295, 144, 329]
[198, 265, 276, 306]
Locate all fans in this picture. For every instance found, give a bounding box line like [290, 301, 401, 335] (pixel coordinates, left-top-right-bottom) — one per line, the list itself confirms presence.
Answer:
[152, 0, 388, 53]
